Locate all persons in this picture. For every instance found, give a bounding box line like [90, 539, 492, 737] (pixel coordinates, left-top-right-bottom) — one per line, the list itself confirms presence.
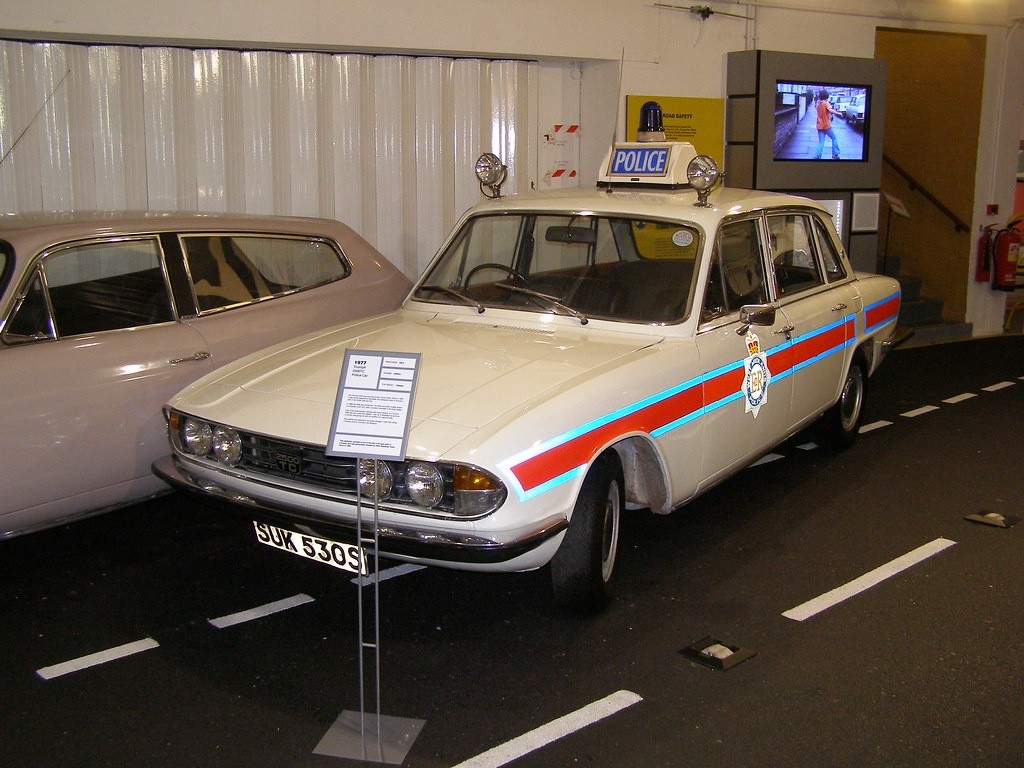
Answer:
[810, 90, 843, 159]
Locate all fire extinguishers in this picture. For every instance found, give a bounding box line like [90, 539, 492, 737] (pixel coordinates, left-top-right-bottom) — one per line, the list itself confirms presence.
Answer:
[992, 219, 1024, 290]
[976, 222, 998, 282]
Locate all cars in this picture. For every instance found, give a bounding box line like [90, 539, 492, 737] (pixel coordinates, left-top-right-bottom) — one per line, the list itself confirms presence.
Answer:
[828, 95, 839, 110]
[0, 207, 415, 539]
[149, 135, 903, 614]
[846, 94, 866, 127]
[835, 97, 852, 116]
